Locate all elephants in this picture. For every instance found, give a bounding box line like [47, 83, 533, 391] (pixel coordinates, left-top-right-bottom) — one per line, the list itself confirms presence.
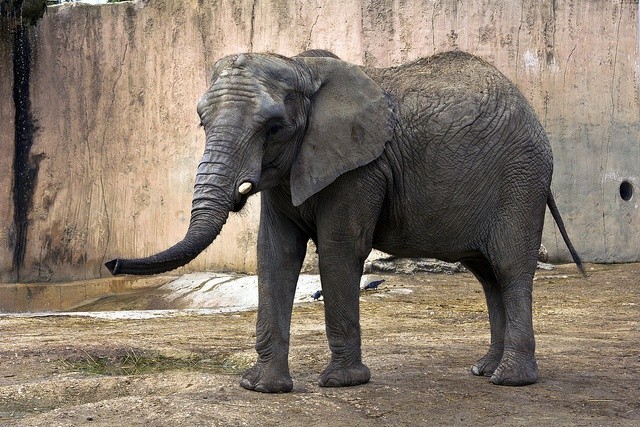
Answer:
[104, 49, 587, 392]
